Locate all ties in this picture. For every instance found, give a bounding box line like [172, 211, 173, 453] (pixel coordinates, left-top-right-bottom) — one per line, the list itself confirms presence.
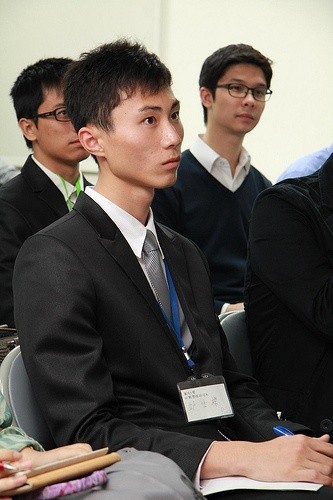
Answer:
[141, 228, 174, 331]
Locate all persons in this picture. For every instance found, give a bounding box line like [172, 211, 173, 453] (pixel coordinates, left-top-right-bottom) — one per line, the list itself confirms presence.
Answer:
[0, 55, 93, 336]
[152, 42, 276, 240]
[0, 366, 91, 495]
[10, 38, 333, 500]
[242, 147, 332, 427]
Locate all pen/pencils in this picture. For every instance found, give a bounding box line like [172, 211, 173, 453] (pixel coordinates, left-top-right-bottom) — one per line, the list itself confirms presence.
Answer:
[273, 426, 294, 436]
[0, 462, 17, 471]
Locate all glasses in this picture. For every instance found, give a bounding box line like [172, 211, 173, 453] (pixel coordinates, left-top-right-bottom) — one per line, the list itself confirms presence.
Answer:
[217, 83, 273, 102]
[30, 107, 73, 123]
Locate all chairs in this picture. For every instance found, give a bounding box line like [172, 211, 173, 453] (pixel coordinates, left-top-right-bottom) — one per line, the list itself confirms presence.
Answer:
[0, 345, 54, 453]
[217, 311, 247, 377]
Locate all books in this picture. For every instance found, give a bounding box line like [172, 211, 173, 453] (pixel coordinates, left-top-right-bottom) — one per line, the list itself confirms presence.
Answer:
[0, 447, 120, 500]
